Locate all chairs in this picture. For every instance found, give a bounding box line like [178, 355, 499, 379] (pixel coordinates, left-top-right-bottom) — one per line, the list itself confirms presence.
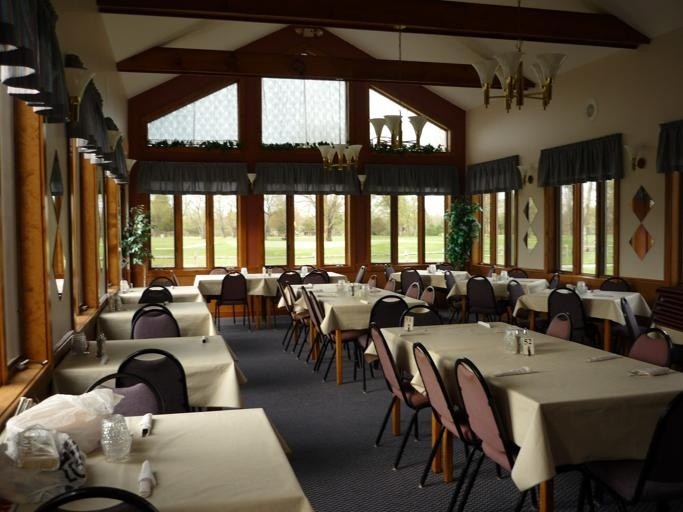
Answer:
[369, 321, 431, 471]
[115, 348, 190, 414]
[454, 358, 595, 511]
[35, 486, 157, 511]
[412, 342, 501, 512]
[85, 373, 164, 416]
[131, 262, 682, 339]
[577, 392, 682, 511]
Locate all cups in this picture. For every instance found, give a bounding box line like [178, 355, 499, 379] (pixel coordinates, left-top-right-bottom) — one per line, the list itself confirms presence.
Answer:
[336, 279, 347, 294]
[69, 329, 88, 356]
[119, 279, 129, 292]
[239, 268, 248, 278]
[299, 265, 309, 275]
[100, 414, 134, 463]
[362, 283, 369, 301]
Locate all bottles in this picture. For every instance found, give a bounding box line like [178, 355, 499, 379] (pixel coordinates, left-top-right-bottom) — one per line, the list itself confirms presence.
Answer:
[262, 265, 271, 276]
[114, 296, 121, 310]
[513, 328, 527, 354]
[108, 295, 114, 310]
[348, 283, 360, 297]
[95, 332, 105, 359]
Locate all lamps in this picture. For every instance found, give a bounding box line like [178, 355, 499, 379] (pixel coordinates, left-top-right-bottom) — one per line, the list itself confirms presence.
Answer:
[125, 159, 138, 175]
[624, 144, 647, 169]
[516, 165, 533, 186]
[63, 66, 97, 123]
[317, 142, 364, 171]
[246, 167, 257, 190]
[357, 174, 369, 194]
[105, 129, 125, 151]
[366, 24, 429, 155]
[470, 0, 568, 113]
